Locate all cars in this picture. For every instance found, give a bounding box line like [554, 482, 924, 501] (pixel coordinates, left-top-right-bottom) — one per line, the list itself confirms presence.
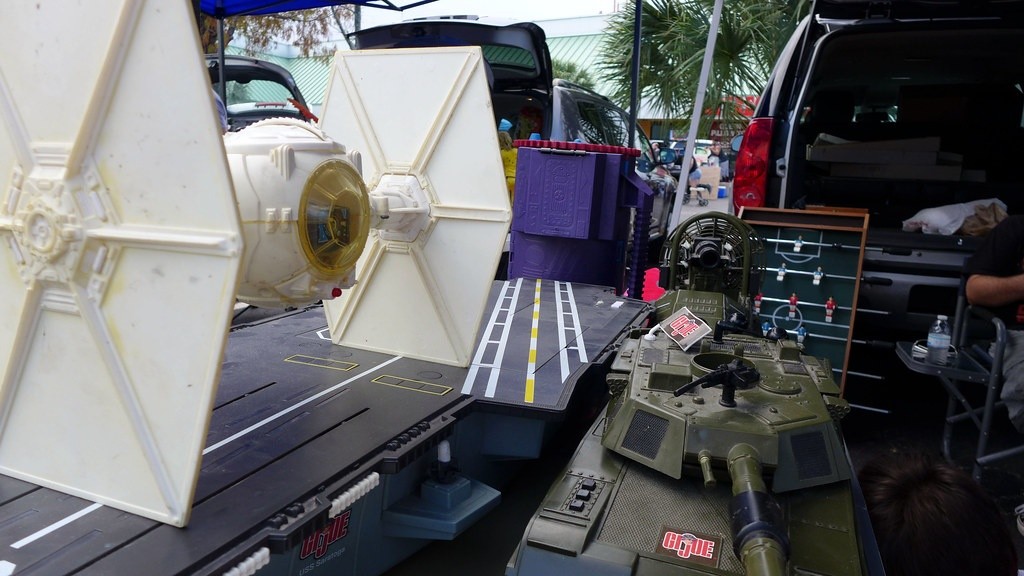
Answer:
[650, 138, 731, 183]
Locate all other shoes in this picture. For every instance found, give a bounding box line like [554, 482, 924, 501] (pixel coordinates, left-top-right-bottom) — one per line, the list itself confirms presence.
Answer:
[1015, 504, 1024, 537]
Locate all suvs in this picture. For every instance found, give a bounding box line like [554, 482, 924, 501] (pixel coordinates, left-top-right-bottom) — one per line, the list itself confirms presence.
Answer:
[208, 53, 318, 131]
[731, 0, 1024, 454]
[346, 15, 679, 270]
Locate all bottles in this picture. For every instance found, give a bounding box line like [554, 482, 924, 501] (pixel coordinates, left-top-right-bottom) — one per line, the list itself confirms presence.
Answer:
[928, 314, 953, 367]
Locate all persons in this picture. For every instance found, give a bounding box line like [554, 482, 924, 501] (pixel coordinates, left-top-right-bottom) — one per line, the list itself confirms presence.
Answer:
[497, 118, 513, 151]
[965, 214, 1024, 536]
[652, 142, 667, 169]
[854, 452, 1019, 576]
[706, 138, 730, 182]
[688, 156, 703, 200]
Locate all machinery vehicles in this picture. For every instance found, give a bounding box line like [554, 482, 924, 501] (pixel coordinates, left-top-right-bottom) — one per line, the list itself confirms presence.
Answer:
[504, 317, 887, 576]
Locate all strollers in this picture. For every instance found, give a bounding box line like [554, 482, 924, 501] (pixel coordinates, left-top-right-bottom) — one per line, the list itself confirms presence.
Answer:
[682, 186, 709, 206]
